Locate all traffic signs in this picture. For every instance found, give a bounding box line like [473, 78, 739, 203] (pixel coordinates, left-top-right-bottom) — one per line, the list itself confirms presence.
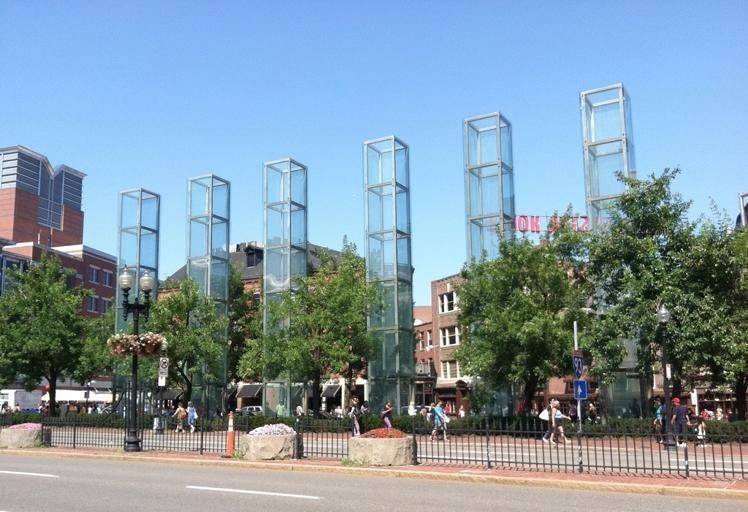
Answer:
[572, 350, 583, 379]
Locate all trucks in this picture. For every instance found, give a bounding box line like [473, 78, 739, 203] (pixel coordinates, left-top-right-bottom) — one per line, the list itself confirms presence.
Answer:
[0, 388, 43, 415]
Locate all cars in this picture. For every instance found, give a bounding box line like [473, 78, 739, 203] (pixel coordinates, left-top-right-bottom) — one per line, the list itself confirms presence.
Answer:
[239, 405, 263, 414]
[687, 408, 705, 421]
[309, 409, 338, 419]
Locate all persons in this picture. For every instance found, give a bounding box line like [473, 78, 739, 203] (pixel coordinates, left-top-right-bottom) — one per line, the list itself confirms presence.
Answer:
[274, 400, 304, 423]
[170, 401, 197, 434]
[1, 397, 111, 417]
[348, 398, 466, 442]
[537, 395, 732, 449]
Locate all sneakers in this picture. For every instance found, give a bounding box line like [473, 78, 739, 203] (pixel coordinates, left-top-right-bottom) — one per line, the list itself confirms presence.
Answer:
[175, 427, 195, 434]
[542, 437, 572, 445]
[676, 441, 688, 448]
[697, 444, 707, 448]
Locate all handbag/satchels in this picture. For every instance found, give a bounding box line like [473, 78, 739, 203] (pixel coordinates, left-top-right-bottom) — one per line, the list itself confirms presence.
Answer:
[538, 404, 552, 422]
[193, 408, 199, 420]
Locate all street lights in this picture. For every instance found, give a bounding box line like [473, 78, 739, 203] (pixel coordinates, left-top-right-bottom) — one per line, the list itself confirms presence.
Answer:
[656, 301, 677, 452]
[85, 379, 96, 415]
[117, 262, 155, 454]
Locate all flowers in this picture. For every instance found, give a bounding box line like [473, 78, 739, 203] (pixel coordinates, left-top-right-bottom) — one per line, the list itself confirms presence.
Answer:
[107, 331, 168, 356]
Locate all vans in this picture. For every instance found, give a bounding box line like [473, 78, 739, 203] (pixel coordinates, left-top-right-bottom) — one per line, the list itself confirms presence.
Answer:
[402, 404, 459, 421]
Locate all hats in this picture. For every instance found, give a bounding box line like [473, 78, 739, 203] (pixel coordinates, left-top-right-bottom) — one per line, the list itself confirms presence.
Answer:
[671, 397, 680, 403]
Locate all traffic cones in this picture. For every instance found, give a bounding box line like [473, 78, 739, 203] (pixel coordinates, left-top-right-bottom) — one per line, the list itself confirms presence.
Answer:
[219, 410, 242, 459]
